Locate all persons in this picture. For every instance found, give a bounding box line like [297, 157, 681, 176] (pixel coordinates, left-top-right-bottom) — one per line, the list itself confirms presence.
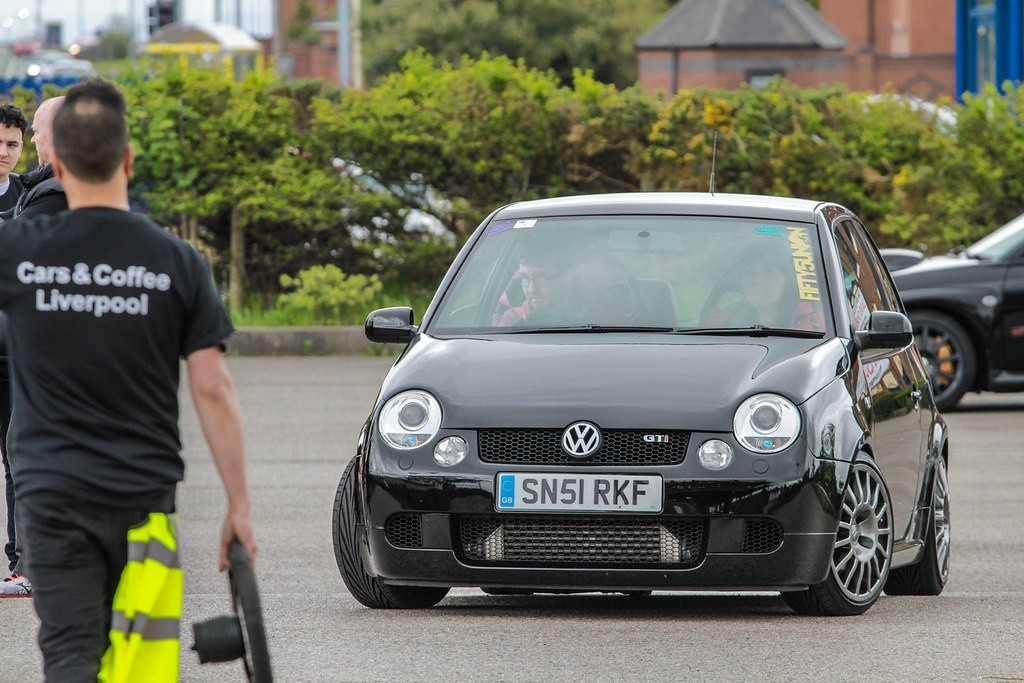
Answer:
[0, 95, 68, 599]
[499, 254, 574, 327]
[706, 263, 826, 333]
[0, 77, 258, 683]
[0, 105, 28, 214]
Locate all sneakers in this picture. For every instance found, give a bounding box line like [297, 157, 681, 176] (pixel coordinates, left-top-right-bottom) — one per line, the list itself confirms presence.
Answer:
[0, 574, 34, 599]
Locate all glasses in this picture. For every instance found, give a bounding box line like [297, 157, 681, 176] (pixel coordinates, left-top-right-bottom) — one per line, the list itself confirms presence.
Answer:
[518, 269, 564, 288]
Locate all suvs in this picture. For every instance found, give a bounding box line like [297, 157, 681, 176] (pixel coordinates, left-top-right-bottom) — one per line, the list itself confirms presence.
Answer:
[878, 212, 1024, 413]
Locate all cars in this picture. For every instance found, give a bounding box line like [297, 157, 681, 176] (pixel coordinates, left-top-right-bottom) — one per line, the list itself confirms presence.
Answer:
[331, 132, 951, 616]
